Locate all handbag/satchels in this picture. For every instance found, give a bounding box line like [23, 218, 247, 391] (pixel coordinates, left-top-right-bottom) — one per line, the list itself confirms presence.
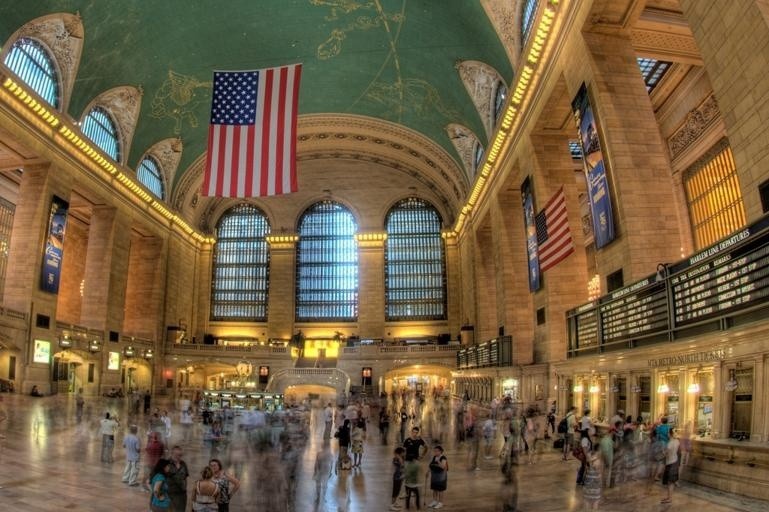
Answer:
[335, 432, 340, 437]
[572, 446, 586, 461]
[340, 458, 350, 470]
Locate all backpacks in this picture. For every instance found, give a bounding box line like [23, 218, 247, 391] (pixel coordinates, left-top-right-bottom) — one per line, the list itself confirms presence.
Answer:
[557, 414, 573, 433]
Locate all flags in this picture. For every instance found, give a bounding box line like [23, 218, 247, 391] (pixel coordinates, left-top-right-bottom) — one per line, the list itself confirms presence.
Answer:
[536, 187, 574, 273]
[202, 65, 300, 197]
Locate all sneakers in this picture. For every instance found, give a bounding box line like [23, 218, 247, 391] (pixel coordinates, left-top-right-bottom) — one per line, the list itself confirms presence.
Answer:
[433, 503, 443, 509]
[428, 501, 437, 508]
[389, 493, 407, 511]
[129, 483, 138, 486]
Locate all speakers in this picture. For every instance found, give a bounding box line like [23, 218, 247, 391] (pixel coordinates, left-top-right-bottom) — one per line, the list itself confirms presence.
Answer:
[204, 334, 214, 346]
[437, 333, 449, 345]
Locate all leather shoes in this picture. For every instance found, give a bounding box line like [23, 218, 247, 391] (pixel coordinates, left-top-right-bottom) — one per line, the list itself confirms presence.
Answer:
[660, 497, 671, 502]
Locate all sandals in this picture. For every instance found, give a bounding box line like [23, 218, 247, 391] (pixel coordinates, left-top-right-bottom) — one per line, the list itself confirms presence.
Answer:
[654, 476, 660, 480]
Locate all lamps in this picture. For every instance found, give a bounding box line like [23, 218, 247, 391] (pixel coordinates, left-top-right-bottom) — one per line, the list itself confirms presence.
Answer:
[655, 260, 672, 283]
[686, 363, 702, 393]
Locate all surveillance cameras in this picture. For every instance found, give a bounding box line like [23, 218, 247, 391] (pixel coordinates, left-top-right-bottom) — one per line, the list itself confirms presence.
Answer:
[656, 274, 662, 283]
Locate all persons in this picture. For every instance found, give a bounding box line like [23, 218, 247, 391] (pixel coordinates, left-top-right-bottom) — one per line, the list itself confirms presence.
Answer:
[29, 386, 679, 512]
